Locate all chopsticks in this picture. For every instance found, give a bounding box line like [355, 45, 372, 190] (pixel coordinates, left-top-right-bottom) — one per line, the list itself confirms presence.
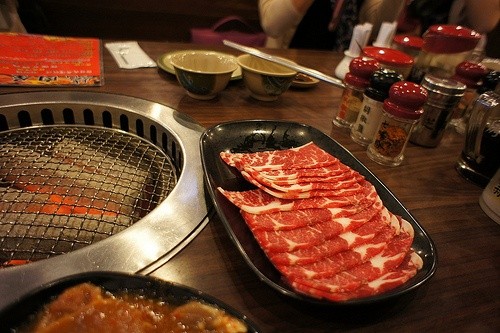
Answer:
[223, 39, 346, 89]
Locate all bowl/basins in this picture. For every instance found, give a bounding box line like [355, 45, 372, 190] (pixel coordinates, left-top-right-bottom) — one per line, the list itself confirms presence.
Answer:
[236, 54, 299, 102]
[169, 51, 239, 101]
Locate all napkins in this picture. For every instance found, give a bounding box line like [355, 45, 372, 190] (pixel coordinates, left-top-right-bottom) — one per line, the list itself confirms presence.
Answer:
[103, 40, 158, 69]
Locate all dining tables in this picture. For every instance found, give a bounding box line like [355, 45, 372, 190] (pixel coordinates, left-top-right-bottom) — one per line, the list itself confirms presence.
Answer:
[0, 39, 500, 333]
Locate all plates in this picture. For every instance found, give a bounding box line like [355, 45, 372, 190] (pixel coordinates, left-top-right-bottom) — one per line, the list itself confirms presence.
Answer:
[290, 73, 320, 87]
[157, 49, 243, 81]
[199, 119, 439, 308]
[0, 271, 263, 333]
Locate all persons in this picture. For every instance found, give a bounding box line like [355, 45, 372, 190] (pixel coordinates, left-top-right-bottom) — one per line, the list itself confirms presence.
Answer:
[258, 0, 500, 51]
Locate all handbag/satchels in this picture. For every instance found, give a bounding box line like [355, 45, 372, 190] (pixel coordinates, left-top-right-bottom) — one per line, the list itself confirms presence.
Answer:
[191, 15, 266, 49]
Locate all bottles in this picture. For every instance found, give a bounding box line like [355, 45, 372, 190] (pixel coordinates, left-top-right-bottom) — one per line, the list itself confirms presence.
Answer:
[333, 23, 500, 225]
